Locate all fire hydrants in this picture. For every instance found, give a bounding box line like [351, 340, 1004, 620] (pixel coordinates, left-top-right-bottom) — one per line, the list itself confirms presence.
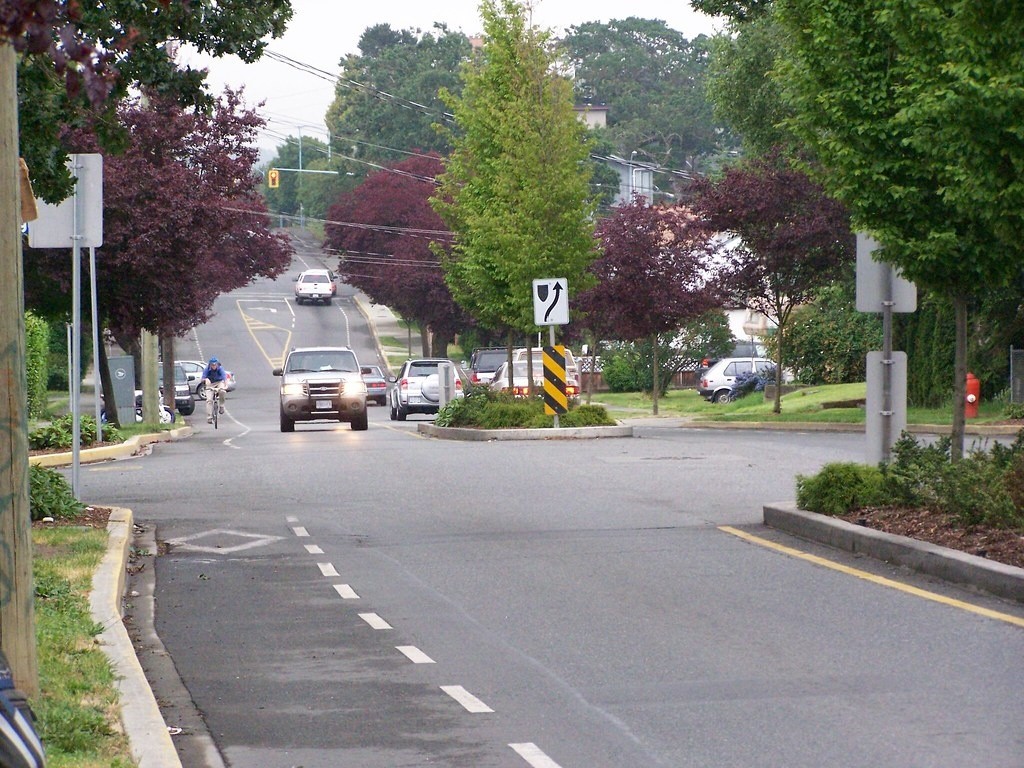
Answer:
[964, 372, 980, 418]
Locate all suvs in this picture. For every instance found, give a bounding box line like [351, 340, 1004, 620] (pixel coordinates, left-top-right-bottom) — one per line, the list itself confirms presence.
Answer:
[509, 346, 581, 388]
[388, 358, 465, 421]
[468, 347, 515, 389]
[292, 272, 334, 306]
[156, 361, 195, 416]
[273, 345, 371, 433]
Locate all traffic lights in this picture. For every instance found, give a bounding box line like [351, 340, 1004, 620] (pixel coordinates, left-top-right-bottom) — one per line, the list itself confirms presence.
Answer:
[268, 169, 279, 188]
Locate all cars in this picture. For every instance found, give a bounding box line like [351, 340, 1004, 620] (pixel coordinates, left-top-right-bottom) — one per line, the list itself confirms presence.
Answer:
[177, 358, 236, 400]
[306, 269, 339, 296]
[359, 365, 387, 406]
[490, 360, 580, 402]
[695, 358, 808, 404]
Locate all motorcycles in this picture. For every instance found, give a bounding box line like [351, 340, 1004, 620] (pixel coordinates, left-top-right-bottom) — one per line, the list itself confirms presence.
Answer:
[100, 386, 175, 428]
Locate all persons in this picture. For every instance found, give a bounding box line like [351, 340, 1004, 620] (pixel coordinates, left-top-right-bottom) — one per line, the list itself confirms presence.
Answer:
[201, 357, 227, 424]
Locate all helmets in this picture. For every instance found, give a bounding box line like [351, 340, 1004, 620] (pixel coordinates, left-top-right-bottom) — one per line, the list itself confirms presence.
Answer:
[209, 356, 218, 363]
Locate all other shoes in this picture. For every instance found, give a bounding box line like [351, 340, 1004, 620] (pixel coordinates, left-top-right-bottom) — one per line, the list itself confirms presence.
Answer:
[208, 418, 213, 424]
[219, 407, 224, 414]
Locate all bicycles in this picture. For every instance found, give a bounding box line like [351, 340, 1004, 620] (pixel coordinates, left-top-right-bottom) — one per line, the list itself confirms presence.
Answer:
[203, 387, 226, 429]
